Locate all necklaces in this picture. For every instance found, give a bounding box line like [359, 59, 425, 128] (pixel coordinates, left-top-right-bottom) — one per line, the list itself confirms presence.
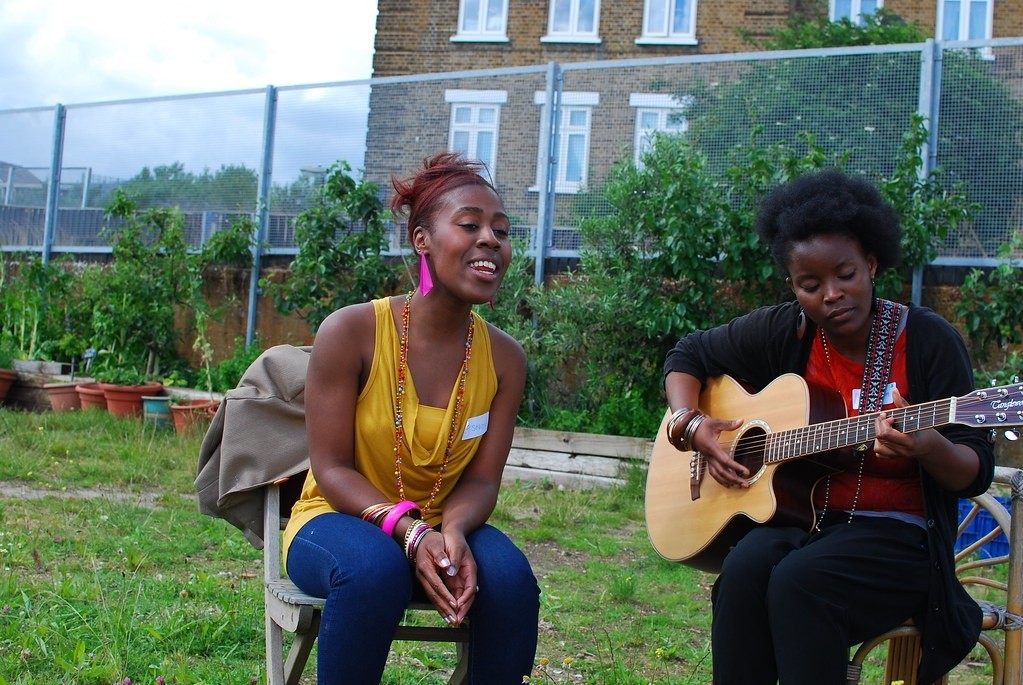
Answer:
[396, 288, 475, 524]
[816, 325, 863, 533]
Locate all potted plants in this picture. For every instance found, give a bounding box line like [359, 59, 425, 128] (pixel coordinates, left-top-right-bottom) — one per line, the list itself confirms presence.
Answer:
[0, 331, 17, 406]
[164, 393, 220, 437]
[44, 376, 82, 414]
[98, 364, 162, 421]
[9, 254, 85, 375]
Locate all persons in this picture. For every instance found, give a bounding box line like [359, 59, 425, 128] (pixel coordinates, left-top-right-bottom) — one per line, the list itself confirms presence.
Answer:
[283, 152, 542, 685]
[662, 165, 997, 685]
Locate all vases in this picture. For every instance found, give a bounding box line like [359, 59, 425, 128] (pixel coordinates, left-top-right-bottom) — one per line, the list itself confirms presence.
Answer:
[208, 405, 219, 424]
[75, 383, 106, 412]
[141, 396, 174, 429]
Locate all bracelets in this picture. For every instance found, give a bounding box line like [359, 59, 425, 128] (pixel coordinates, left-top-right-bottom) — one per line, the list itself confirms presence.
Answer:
[666, 407, 707, 452]
[361, 499, 435, 561]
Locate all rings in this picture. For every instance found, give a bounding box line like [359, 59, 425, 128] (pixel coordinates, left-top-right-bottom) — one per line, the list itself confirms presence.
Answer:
[476, 585, 479, 592]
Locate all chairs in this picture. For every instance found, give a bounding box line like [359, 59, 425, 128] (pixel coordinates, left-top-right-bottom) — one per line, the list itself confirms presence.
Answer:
[844, 466, 1023, 685]
[264, 345, 471, 685]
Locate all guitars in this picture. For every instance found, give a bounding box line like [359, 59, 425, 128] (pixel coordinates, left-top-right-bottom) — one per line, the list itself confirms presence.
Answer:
[644, 372, 1023, 573]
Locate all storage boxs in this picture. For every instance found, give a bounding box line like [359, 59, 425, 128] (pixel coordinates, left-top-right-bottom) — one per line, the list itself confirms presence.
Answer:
[954, 495, 1013, 560]
